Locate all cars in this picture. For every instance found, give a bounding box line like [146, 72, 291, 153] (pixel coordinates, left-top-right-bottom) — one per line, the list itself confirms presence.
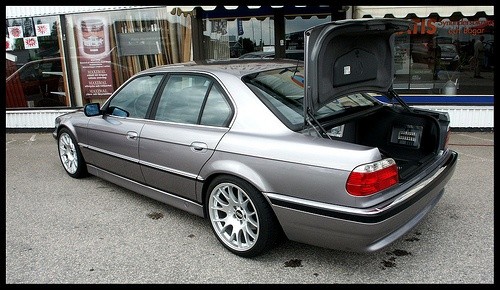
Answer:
[53, 18, 460, 258]
[7, 57, 132, 108]
[428, 37, 460, 71]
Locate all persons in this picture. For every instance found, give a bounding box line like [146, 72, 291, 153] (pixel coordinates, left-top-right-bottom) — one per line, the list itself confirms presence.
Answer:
[45, 52, 63, 97]
[454, 36, 477, 72]
[473, 36, 489, 79]
[427, 36, 442, 81]
